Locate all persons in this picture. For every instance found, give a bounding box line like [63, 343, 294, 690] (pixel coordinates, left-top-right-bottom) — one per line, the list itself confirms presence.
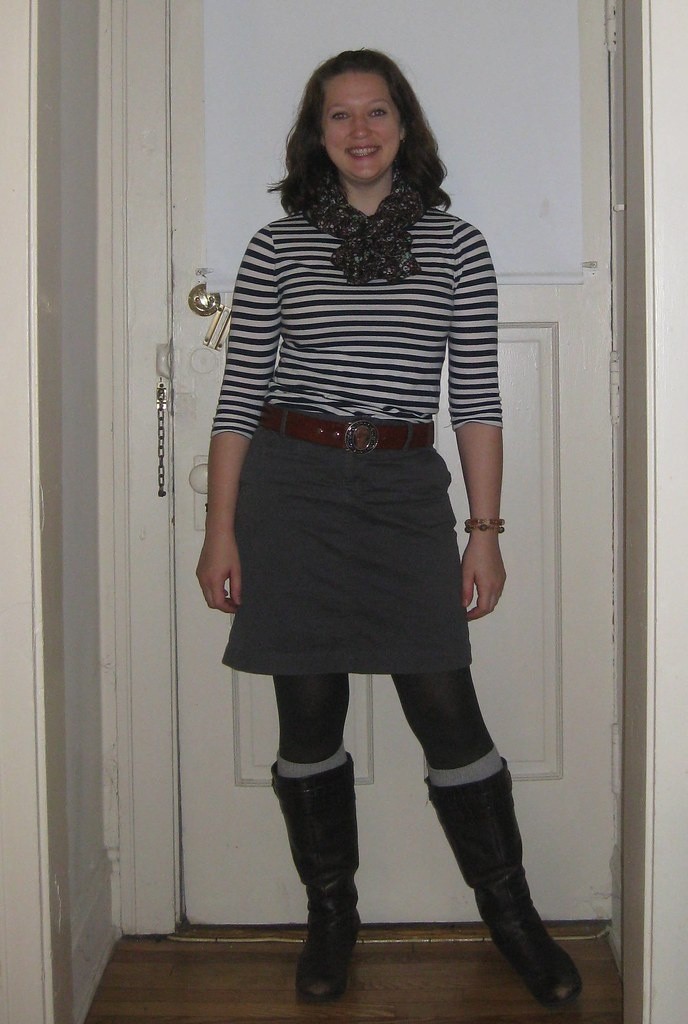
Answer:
[196, 50, 582, 1008]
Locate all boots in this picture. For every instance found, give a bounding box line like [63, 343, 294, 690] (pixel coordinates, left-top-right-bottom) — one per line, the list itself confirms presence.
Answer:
[424, 756, 582, 1008]
[271, 752, 361, 1001]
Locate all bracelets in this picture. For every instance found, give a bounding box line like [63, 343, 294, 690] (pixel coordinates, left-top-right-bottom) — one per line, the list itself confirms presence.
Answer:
[464, 519, 505, 533]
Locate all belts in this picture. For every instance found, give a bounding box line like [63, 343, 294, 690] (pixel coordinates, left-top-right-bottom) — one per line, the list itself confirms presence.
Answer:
[259, 402, 435, 456]
